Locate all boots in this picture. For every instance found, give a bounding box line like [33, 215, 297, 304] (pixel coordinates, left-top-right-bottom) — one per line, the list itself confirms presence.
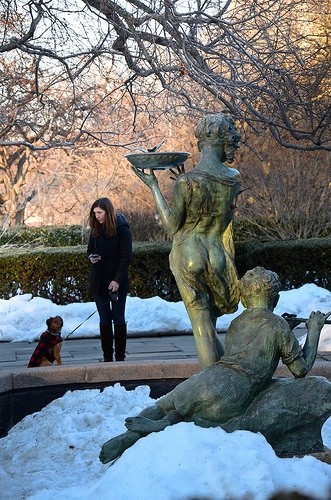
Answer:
[99, 324, 113, 362]
[114, 322, 127, 361]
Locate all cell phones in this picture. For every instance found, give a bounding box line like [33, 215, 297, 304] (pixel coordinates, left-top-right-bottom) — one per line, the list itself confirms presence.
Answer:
[92, 254, 99, 258]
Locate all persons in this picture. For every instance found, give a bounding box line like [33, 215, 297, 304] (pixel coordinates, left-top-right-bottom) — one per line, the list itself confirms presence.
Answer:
[87, 198, 133, 363]
[131, 114, 241, 369]
[98, 266, 331, 465]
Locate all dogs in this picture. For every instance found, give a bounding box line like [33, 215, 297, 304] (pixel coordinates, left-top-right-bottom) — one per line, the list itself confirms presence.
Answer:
[27, 315, 64, 368]
